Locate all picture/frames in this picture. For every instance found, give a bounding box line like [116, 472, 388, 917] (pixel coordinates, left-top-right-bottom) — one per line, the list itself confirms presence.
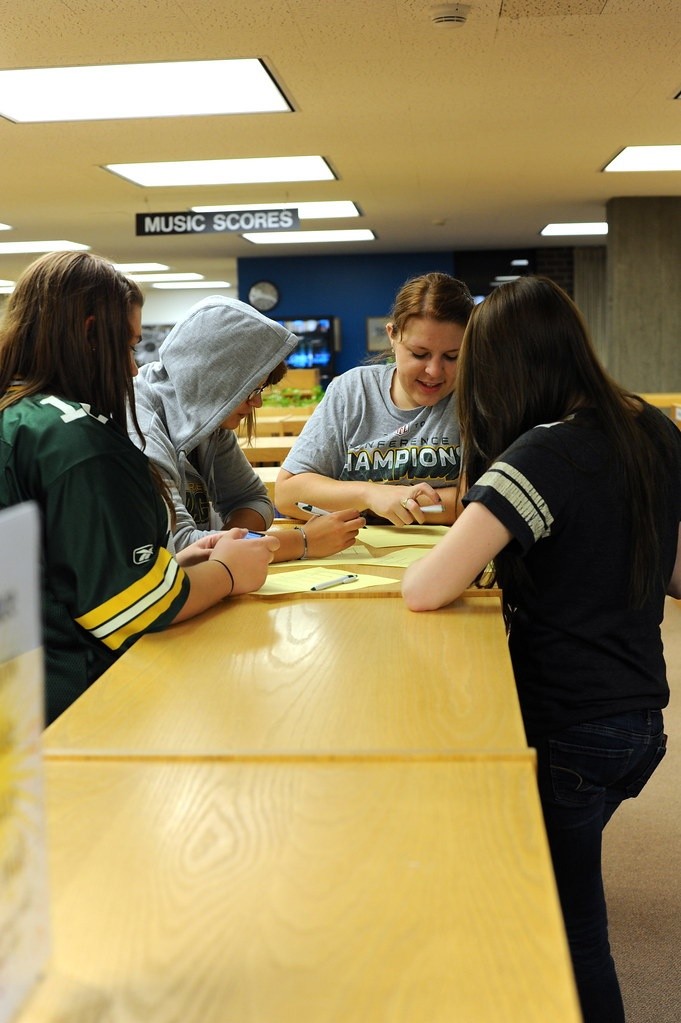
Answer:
[367, 317, 392, 352]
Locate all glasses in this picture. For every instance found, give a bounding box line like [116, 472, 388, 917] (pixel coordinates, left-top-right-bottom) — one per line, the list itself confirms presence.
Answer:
[247, 383, 269, 401]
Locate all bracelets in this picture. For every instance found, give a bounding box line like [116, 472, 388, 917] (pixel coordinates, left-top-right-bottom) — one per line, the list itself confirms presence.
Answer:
[294, 526, 307, 561]
[208, 559, 234, 600]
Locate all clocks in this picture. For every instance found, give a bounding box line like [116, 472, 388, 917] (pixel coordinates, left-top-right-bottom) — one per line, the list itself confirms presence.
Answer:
[248, 280, 279, 312]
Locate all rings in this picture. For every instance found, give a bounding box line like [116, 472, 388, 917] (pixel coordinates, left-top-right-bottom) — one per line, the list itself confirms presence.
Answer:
[402, 497, 414, 508]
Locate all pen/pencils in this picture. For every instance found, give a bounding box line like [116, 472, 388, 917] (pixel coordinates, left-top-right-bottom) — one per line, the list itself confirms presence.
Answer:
[419, 504, 445, 513]
[293, 501, 368, 530]
[308, 572, 358, 592]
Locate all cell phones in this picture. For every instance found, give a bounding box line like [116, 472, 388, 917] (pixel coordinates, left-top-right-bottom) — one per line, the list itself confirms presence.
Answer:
[245, 531, 267, 539]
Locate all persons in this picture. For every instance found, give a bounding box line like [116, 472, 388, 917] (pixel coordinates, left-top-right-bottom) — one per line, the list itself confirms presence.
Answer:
[0, 248, 280, 738]
[275, 271, 477, 529]
[401, 270, 681, 1023]
[123, 293, 366, 568]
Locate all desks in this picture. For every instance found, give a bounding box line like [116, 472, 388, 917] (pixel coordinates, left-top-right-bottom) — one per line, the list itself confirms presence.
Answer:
[14, 392, 681, 1023]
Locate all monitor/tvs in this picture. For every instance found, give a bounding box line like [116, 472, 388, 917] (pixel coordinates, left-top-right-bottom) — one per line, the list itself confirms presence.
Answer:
[266, 315, 336, 380]
